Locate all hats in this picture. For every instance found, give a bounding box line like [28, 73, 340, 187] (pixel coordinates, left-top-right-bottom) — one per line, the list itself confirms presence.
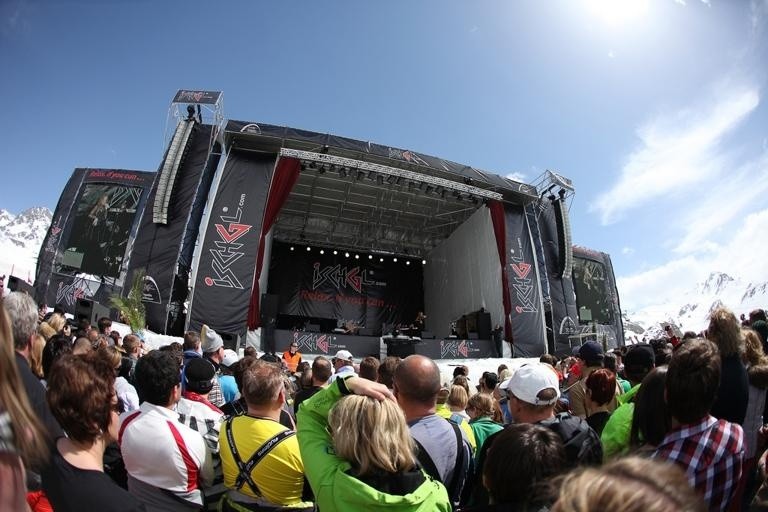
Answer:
[439, 372, 452, 399]
[201, 324, 224, 353]
[499, 363, 561, 405]
[625, 344, 655, 375]
[572, 341, 602, 361]
[222, 349, 238, 367]
[332, 350, 353, 362]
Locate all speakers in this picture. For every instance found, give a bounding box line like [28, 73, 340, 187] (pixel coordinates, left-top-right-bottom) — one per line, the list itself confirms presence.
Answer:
[214, 329, 237, 352]
[468, 332, 480, 340]
[75, 298, 110, 328]
[357, 328, 376, 337]
[152, 120, 197, 227]
[477, 313, 491, 340]
[553, 199, 573, 280]
[305, 324, 322, 332]
[420, 331, 433, 338]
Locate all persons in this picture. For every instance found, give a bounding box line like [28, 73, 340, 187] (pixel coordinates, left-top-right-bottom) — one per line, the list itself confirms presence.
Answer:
[0, 290, 768, 512]
[87, 194, 126, 244]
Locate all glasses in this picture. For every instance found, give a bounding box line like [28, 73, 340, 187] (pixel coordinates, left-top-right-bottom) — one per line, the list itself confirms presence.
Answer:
[112, 398, 124, 412]
